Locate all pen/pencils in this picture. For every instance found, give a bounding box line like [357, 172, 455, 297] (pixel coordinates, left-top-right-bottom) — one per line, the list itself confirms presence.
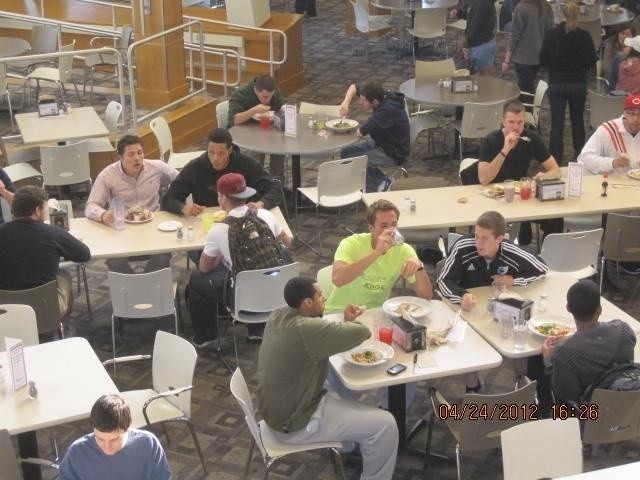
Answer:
[413, 354, 418, 374]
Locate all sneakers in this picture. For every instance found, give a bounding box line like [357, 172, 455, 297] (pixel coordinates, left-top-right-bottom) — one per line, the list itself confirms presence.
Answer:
[610, 261, 639, 275]
[191, 333, 217, 347]
[247, 325, 265, 341]
[340, 441, 361, 459]
[465, 379, 482, 395]
[377, 177, 395, 192]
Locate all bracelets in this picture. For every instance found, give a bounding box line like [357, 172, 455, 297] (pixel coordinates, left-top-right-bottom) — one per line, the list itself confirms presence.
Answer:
[417, 265, 424, 272]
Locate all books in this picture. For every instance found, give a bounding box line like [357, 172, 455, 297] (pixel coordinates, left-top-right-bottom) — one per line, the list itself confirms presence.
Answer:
[567, 162, 583, 198]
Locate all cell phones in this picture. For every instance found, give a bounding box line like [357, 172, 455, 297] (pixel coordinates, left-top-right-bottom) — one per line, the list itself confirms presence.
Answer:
[385, 363, 407, 377]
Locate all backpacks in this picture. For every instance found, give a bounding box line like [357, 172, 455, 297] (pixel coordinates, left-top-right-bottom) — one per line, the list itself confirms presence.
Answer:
[578, 360, 640, 439]
[221, 207, 295, 281]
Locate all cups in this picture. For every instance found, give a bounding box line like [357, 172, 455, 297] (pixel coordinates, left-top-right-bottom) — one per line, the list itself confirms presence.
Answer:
[260, 112, 269, 129]
[520, 177, 530, 201]
[315, 110, 325, 130]
[202, 209, 214, 231]
[390, 229, 404, 246]
[377, 319, 394, 345]
[503, 179, 515, 203]
[488, 279, 528, 350]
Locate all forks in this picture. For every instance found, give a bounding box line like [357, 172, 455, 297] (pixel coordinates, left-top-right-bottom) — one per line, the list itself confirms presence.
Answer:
[520, 136, 530, 143]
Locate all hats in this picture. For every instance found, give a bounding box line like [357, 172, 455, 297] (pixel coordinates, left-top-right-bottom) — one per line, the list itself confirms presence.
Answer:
[217, 173, 257, 199]
[623, 94, 640, 109]
[624, 35, 640, 53]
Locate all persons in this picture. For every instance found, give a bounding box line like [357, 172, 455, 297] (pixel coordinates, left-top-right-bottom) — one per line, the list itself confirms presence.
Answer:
[227, 73, 285, 179]
[324, 199, 433, 411]
[436, 211, 550, 394]
[340, 79, 410, 192]
[185, 171, 294, 347]
[577, 97, 640, 275]
[0, 184, 91, 340]
[256, 277, 399, 480]
[59, 394, 171, 480]
[447, 1, 640, 186]
[84, 135, 176, 273]
[543, 279, 636, 415]
[161, 129, 282, 216]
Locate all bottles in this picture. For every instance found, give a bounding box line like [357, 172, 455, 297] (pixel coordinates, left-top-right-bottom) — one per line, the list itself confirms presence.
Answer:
[177, 224, 194, 241]
[28, 381, 39, 400]
[113, 197, 125, 230]
[601, 173, 608, 197]
[539, 295, 548, 311]
[402, 195, 409, 209]
[308, 116, 314, 129]
[439, 77, 479, 92]
[62, 102, 70, 114]
[410, 200, 416, 214]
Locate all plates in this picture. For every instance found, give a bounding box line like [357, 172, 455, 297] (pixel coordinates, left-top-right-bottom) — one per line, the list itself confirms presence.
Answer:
[502, 181, 537, 192]
[251, 111, 277, 124]
[125, 212, 154, 223]
[344, 342, 395, 367]
[528, 315, 577, 338]
[481, 188, 495, 198]
[382, 296, 433, 318]
[627, 168, 640, 180]
[158, 221, 182, 231]
[325, 119, 359, 132]
